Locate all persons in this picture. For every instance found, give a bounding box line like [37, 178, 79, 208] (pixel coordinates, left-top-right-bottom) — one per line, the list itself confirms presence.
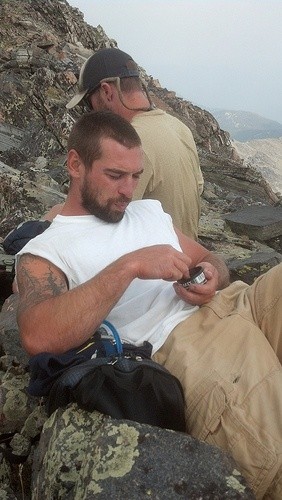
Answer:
[14, 109, 282, 500]
[39, 49, 205, 242]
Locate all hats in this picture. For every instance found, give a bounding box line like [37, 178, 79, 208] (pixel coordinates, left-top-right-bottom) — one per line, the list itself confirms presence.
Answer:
[65, 48, 139, 109]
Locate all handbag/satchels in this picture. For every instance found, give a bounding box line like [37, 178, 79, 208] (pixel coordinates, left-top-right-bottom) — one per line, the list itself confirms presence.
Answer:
[27, 332, 154, 398]
[45, 320, 188, 432]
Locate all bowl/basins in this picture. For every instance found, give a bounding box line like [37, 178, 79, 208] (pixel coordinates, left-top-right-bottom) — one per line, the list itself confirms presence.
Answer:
[177, 266, 207, 291]
